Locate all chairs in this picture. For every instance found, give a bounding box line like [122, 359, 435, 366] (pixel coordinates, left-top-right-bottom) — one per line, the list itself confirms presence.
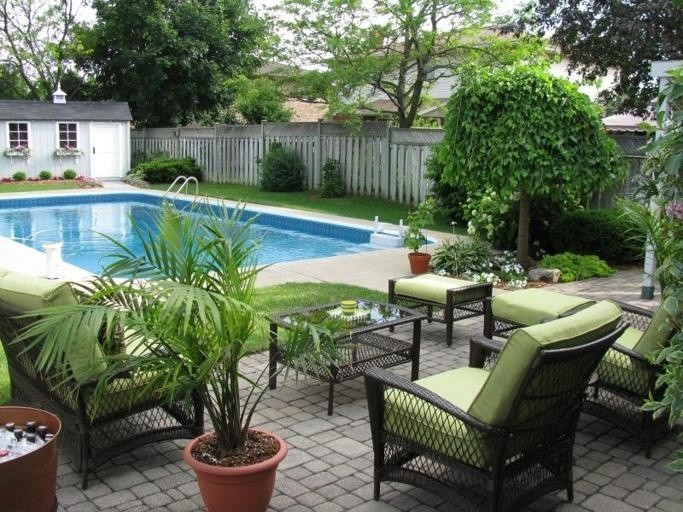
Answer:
[1, 270, 212, 490]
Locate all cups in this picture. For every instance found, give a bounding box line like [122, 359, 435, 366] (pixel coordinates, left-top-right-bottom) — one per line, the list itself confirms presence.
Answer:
[340, 299, 357, 316]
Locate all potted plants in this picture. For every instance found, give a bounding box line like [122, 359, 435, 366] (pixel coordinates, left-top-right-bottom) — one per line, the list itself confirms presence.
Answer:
[10, 197, 294, 511]
[406, 196, 435, 274]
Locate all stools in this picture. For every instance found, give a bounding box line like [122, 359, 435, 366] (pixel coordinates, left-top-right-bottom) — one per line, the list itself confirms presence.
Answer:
[389, 271, 596, 347]
